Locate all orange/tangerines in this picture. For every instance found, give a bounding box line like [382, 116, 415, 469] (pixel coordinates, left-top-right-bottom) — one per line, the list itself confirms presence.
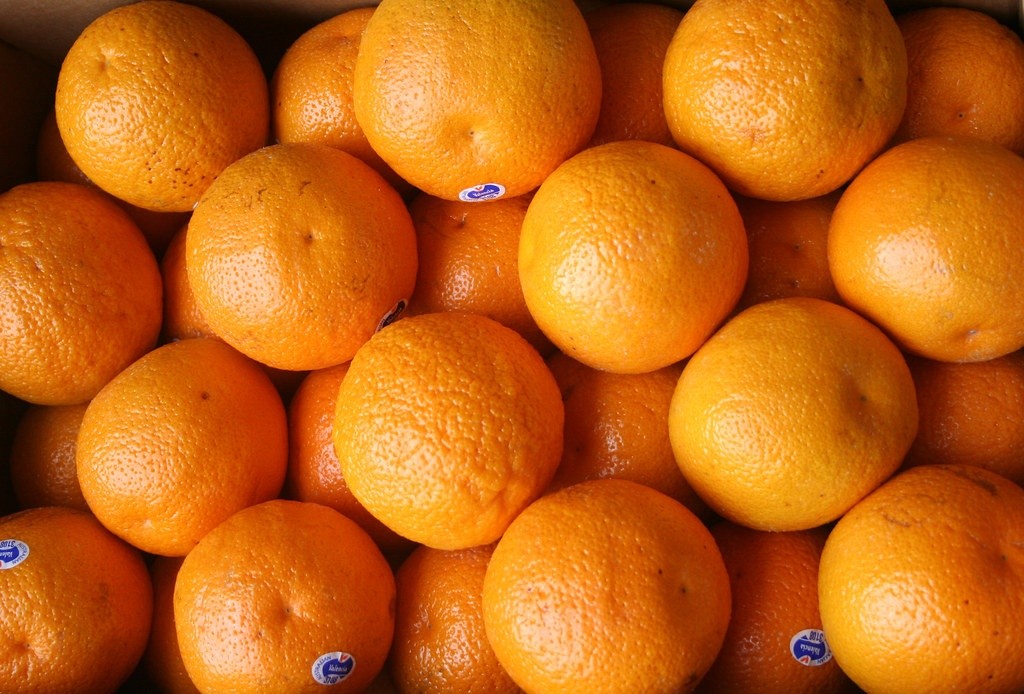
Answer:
[0, 0, 1024, 694]
